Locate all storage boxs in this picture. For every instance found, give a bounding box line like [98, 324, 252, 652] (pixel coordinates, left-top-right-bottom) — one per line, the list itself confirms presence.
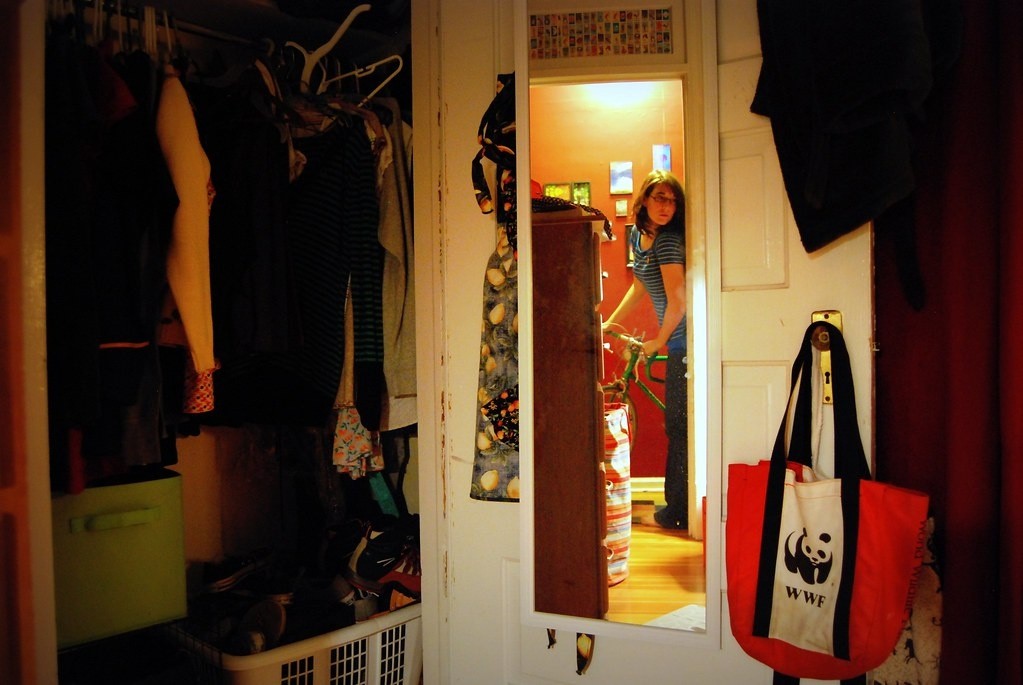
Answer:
[172, 602, 422, 684]
[53, 472, 190, 648]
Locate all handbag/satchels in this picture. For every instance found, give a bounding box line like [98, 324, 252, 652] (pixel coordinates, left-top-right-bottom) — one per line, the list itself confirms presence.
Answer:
[603, 402, 633, 584]
[725, 321, 931, 681]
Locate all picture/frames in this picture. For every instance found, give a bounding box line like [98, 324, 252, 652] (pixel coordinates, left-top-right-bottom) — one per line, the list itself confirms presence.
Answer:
[543, 184, 571, 203]
[652, 145, 671, 171]
[609, 162, 632, 195]
[615, 199, 627, 217]
[571, 182, 590, 217]
[626, 224, 635, 269]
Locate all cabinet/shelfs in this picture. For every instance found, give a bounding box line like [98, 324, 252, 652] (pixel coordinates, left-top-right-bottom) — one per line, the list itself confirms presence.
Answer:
[532, 216, 609, 621]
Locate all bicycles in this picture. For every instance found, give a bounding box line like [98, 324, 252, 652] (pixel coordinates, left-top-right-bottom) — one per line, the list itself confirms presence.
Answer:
[601, 322, 669, 456]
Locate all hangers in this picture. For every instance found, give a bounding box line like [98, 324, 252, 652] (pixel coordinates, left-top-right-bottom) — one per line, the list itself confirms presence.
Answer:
[46, 0, 405, 138]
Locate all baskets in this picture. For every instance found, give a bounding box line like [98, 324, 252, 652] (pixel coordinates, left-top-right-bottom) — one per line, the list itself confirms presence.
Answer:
[160, 602, 424, 685]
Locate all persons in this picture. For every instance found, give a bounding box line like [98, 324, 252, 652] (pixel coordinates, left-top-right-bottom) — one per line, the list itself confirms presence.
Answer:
[600, 168, 687, 532]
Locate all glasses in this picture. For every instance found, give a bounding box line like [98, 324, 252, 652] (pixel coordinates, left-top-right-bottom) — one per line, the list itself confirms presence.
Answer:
[646, 192, 679, 206]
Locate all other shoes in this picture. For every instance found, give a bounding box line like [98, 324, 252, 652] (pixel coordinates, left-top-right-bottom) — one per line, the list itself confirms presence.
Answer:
[183, 513, 421, 655]
[654, 507, 688, 530]
